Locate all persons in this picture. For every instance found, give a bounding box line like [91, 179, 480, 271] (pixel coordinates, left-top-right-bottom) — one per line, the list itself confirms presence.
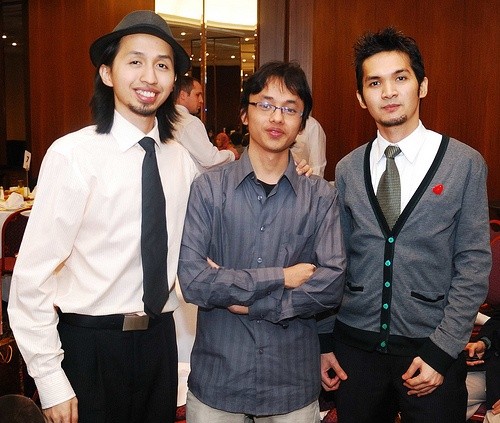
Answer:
[7, 11, 313, 423]
[463, 313, 500, 423]
[216, 132, 233, 152]
[170, 76, 240, 173]
[290, 115, 327, 180]
[320, 28, 493, 423]
[177, 60, 347, 423]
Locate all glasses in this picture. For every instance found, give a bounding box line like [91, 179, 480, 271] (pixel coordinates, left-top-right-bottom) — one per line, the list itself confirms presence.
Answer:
[247, 101, 303, 120]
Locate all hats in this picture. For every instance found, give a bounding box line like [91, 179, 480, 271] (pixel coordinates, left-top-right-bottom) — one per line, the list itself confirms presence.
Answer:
[89, 9, 192, 78]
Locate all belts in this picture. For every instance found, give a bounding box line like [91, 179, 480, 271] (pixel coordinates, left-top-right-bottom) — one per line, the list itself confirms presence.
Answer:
[57, 308, 174, 333]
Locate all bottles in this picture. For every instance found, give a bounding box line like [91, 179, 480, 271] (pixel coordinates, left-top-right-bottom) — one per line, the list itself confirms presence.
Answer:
[0, 186, 4, 200]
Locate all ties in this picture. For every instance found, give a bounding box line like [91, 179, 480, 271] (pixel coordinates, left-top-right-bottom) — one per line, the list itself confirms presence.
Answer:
[139, 137, 169, 318]
[376, 145, 402, 232]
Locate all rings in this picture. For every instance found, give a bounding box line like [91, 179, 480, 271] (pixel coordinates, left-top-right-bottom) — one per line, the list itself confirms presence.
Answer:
[312, 264, 316, 270]
